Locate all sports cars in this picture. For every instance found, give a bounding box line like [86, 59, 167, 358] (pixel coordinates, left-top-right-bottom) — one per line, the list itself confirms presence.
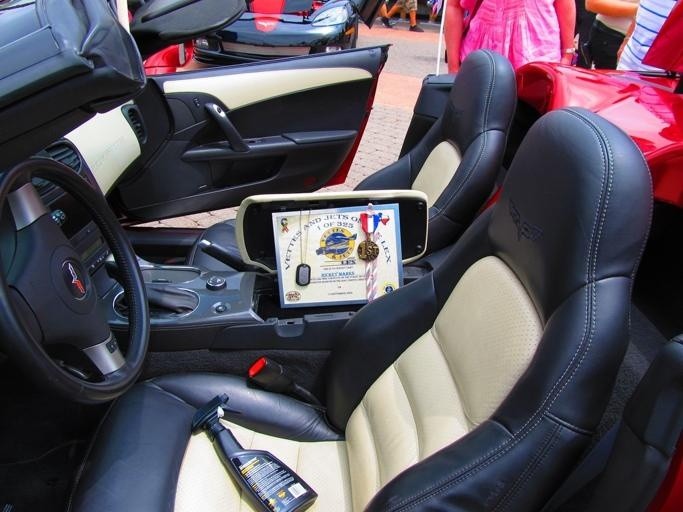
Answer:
[0, 0, 683, 512]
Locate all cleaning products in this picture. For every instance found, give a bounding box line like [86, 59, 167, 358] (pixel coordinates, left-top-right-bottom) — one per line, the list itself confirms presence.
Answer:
[190, 393, 318, 512]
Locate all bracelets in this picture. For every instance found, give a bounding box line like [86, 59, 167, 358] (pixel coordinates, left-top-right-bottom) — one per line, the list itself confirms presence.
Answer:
[624, 36, 631, 40]
[560, 48, 575, 55]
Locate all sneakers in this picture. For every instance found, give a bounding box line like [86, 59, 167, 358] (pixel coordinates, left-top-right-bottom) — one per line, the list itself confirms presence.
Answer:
[380, 15, 393, 28]
[408, 24, 424, 32]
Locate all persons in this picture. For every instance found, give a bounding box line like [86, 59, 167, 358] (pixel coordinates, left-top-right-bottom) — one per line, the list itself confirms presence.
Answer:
[441, 0, 577, 77]
[615, 1, 677, 76]
[385, 1, 424, 32]
[578, 0, 638, 72]
[375, 0, 392, 31]
[426, 0, 443, 23]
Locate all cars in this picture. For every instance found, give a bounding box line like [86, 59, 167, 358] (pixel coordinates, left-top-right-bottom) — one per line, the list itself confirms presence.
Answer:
[189, 0, 388, 67]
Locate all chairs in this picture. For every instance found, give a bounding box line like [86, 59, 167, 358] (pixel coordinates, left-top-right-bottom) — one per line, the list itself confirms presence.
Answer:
[191, 48, 519, 274]
[54, 107, 653, 508]
